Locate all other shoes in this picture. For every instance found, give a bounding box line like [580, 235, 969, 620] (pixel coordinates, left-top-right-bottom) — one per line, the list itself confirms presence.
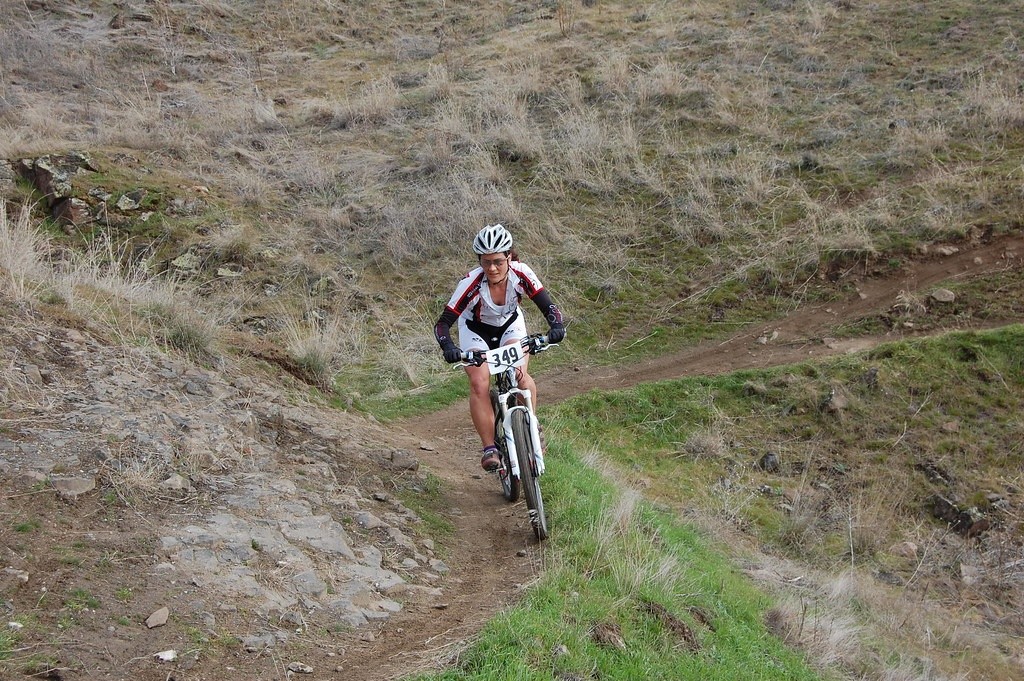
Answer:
[481, 448, 501, 471]
[538, 425, 546, 448]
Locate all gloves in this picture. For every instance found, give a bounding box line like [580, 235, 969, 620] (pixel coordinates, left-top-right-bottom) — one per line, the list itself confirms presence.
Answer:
[546, 323, 564, 345]
[443, 345, 464, 364]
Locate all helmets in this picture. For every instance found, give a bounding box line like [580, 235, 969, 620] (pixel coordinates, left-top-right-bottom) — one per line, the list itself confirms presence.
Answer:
[473, 224, 512, 254]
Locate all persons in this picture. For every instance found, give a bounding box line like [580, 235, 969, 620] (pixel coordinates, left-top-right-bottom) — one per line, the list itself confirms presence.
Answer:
[434, 224, 565, 471]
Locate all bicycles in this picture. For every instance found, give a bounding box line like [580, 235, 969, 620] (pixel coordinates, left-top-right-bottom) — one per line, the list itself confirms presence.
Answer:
[442, 330, 569, 541]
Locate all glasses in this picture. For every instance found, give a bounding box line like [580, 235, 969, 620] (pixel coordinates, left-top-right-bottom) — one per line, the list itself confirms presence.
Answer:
[478, 255, 510, 266]
